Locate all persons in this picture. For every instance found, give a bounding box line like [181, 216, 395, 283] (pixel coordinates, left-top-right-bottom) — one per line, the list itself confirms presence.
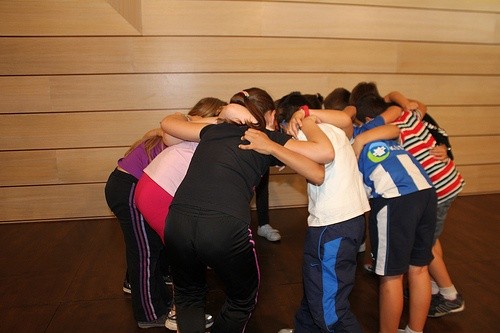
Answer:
[351, 92, 466, 317]
[287, 104, 437, 333]
[254, 91, 301, 243]
[239, 95, 371, 333]
[161, 87, 335, 333]
[135, 102, 259, 331]
[302, 81, 454, 301]
[105, 97, 228, 328]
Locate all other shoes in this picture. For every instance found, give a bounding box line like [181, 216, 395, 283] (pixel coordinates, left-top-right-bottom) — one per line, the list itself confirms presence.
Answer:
[123, 281, 131, 293]
[138, 311, 169, 327]
[358, 243, 366, 252]
[163, 275, 173, 285]
[279, 328, 295, 333]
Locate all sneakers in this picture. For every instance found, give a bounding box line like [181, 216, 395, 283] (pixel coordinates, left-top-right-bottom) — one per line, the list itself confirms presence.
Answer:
[165, 310, 214, 330]
[428, 293, 465, 317]
[404, 288, 409, 298]
[257, 224, 281, 242]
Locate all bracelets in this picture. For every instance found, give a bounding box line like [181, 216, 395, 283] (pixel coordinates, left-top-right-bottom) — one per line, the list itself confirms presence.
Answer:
[299, 105, 309, 119]
[183, 114, 191, 121]
